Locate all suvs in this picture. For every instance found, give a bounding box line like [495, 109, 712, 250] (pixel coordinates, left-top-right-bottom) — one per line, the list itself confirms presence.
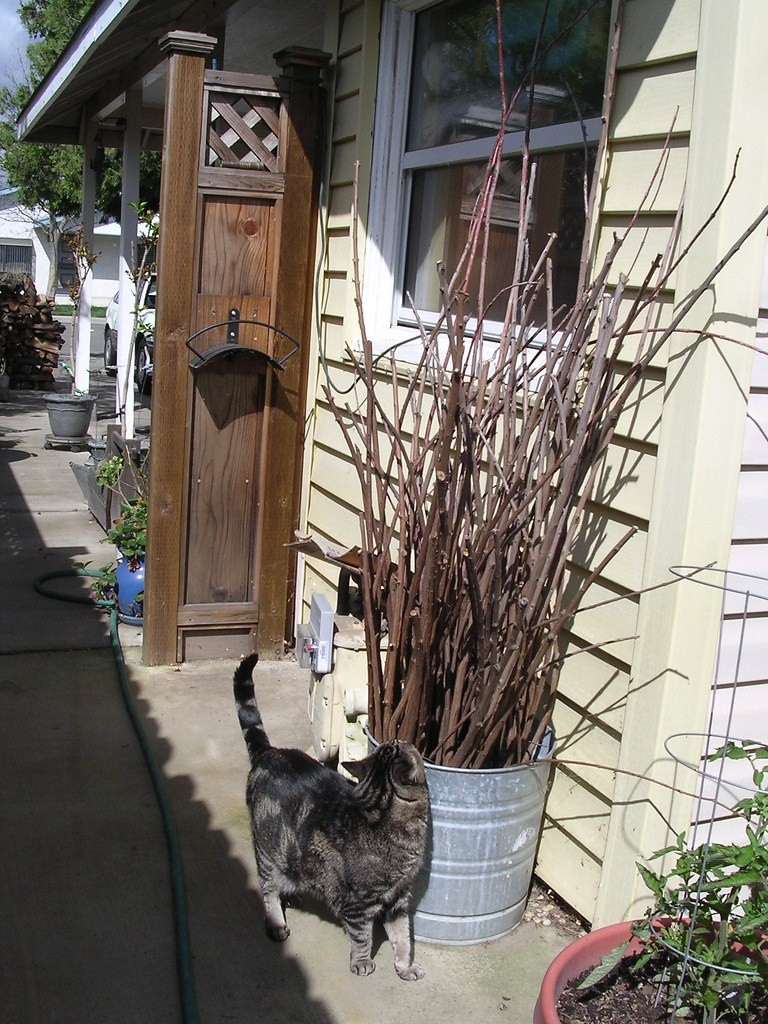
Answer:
[104, 271, 159, 394]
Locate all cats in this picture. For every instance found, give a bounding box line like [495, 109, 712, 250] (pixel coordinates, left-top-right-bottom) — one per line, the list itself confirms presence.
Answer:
[230, 652, 432, 984]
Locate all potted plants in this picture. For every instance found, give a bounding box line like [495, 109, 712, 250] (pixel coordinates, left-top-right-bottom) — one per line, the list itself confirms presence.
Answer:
[535, 738, 768, 1024]
[108, 496, 150, 625]
[42, 392, 99, 436]
